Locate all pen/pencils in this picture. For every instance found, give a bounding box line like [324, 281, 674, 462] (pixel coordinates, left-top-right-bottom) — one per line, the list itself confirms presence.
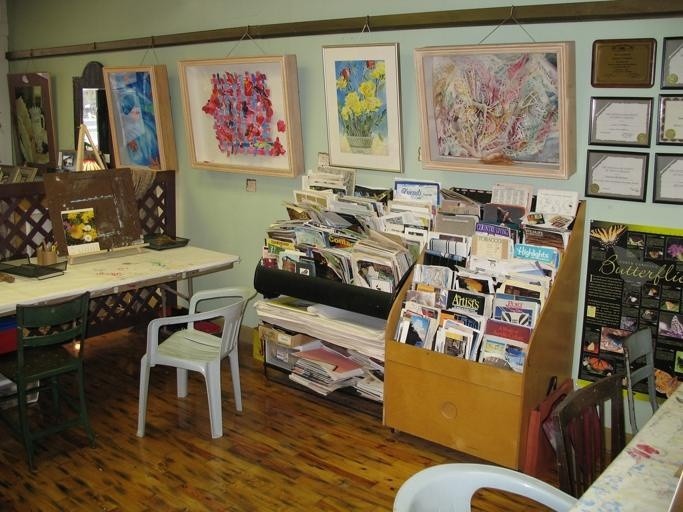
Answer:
[39, 240, 58, 252]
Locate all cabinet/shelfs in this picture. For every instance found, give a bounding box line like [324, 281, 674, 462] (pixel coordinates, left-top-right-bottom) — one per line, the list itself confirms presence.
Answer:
[254, 188, 435, 418]
[380, 191, 587, 472]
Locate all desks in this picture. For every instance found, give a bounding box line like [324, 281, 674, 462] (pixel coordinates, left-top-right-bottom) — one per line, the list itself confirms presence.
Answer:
[572, 379, 683, 512]
[0, 241, 242, 371]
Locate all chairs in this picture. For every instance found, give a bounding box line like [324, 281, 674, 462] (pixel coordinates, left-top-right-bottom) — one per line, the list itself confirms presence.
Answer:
[135, 286, 257, 439]
[0, 291, 92, 472]
[391, 463, 576, 512]
[623, 326, 656, 436]
[552, 374, 624, 499]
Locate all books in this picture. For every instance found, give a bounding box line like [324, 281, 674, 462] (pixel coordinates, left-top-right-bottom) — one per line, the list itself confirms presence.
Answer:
[395, 183, 580, 372]
[254, 294, 387, 402]
[260, 165, 439, 292]
[1, 263, 64, 280]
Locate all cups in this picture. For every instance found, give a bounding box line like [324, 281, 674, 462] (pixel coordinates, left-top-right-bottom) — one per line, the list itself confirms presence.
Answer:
[36, 249, 57, 268]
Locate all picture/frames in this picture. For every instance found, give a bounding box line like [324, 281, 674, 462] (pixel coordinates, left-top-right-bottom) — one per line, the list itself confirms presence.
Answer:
[590, 39, 655, 88]
[583, 150, 649, 203]
[7, 73, 58, 169]
[100, 65, 176, 174]
[176, 53, 304, 179]
[588, 96, 653, 148]
[320, 43, 403, 175]
[652, 36, 683, 206]
[412, 42, 577, 181]
